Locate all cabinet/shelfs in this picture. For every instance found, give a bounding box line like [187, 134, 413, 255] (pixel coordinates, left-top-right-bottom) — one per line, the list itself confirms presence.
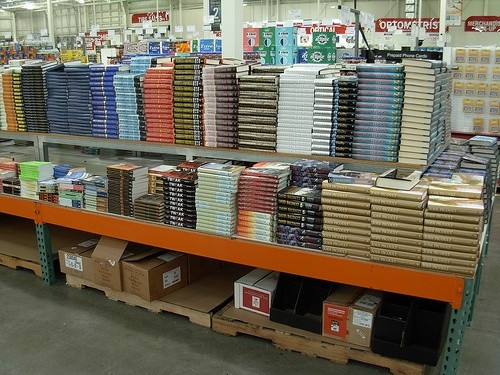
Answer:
[1, 31, 500, 375]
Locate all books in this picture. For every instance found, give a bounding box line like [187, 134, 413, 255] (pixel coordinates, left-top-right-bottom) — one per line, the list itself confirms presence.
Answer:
[0, 55, 500, 279]
